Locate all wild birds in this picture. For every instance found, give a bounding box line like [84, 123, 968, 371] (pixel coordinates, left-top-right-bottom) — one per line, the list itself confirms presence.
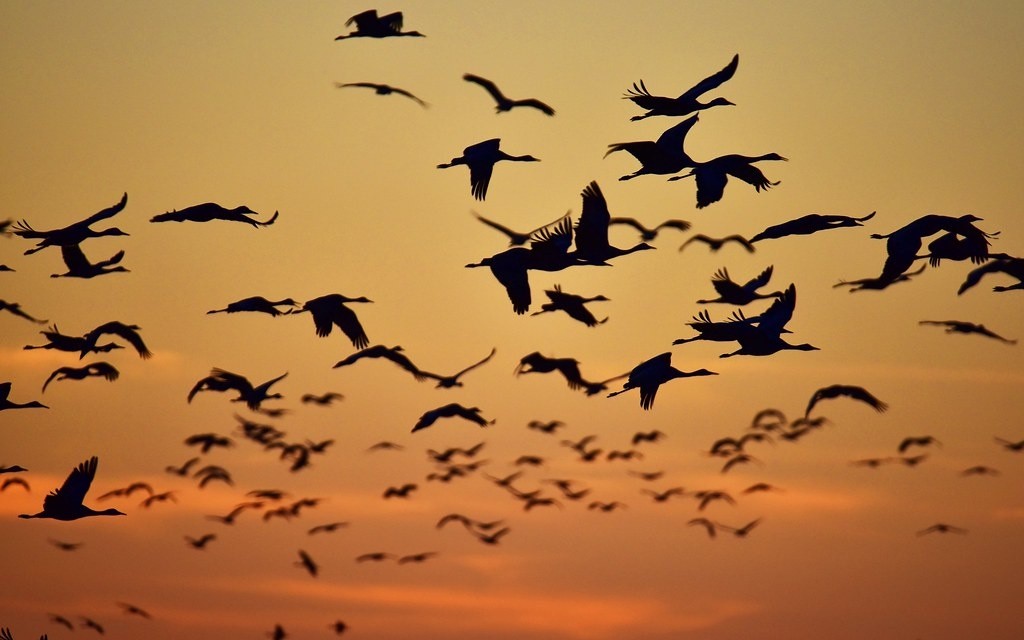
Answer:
[1, 0, 1023, 639]
